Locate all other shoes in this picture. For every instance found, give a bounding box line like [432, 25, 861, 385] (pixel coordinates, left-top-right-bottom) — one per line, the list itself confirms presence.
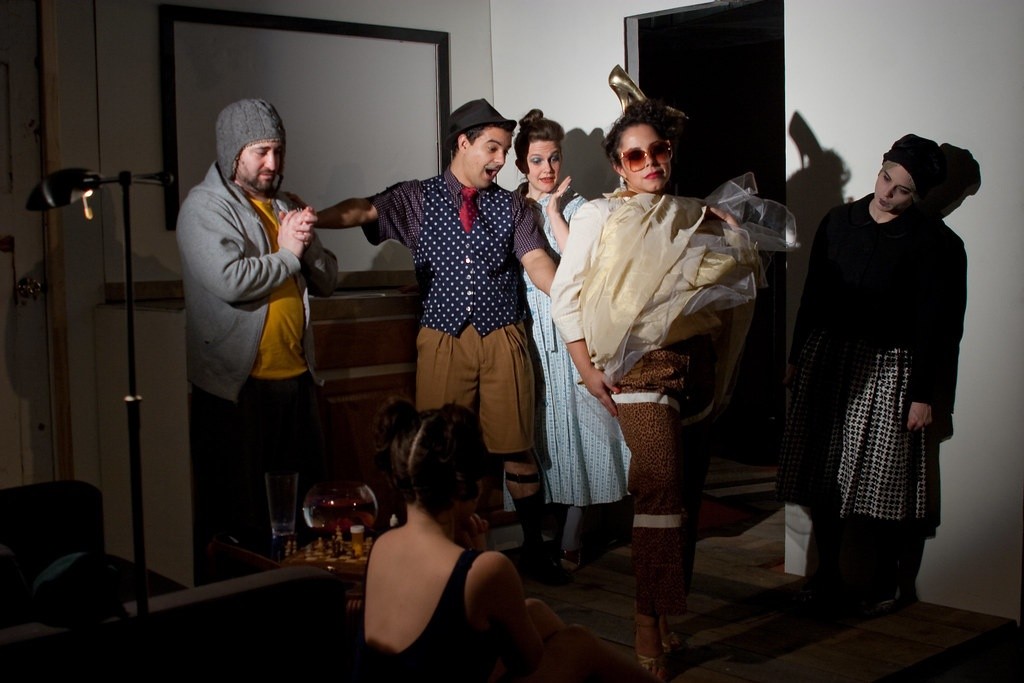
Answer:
[518, 553, 574, 586]
[852, 581, 915, 619]
[554, 523, 583, 573]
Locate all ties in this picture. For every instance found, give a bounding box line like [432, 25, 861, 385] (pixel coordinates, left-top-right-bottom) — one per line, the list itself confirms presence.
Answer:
[458, 186, 479, 234]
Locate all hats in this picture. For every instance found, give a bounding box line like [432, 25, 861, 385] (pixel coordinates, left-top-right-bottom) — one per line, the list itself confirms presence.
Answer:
[444, 98, 517, 151]
[214, 98, 286, 193]
[883, 134, 947, 194]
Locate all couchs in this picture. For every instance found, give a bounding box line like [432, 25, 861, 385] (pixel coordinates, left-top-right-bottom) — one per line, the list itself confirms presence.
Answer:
[0, 480, 334, 683]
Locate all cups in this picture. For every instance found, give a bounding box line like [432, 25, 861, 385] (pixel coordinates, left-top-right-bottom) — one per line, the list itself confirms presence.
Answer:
[265, 471, 298, 539]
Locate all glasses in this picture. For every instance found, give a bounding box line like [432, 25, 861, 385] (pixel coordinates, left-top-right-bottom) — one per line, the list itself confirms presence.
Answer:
[616, 139, 673, 172]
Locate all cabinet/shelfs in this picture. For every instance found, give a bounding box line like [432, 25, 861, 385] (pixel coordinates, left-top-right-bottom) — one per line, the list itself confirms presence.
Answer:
[304, 288, 518, 529]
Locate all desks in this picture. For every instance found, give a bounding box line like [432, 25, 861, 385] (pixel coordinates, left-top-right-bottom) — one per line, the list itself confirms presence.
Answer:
[209, 528, 372, 615]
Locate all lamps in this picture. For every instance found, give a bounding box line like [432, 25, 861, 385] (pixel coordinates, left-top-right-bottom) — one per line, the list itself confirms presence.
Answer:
[25, 169, 157, 630]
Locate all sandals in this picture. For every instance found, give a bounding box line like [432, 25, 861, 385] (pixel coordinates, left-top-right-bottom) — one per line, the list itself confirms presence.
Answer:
[635, 613, 667, 681]
[660, 615, 687, 652]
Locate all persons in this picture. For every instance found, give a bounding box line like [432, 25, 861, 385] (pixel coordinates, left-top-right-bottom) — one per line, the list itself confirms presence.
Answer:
[280, 98, 797, 681]
[776, 134, 966, 618]
[177, 98, 339, 561]
[353, 398, 667, 683]
